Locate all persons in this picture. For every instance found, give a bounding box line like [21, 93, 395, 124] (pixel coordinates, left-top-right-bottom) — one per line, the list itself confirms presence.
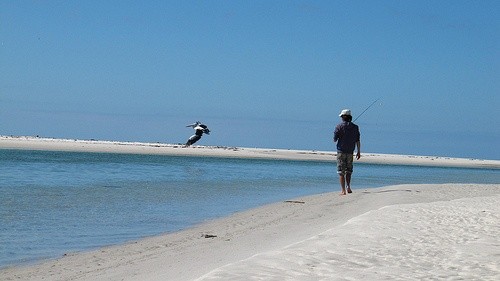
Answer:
[334, 109, 360, 195]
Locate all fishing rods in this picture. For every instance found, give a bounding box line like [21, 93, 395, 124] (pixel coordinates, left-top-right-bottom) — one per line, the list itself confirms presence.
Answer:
[351, 95, 384, 123]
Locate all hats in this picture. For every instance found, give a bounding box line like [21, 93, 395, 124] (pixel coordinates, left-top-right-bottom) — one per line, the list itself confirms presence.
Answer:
[339, 110, 351, 116]
